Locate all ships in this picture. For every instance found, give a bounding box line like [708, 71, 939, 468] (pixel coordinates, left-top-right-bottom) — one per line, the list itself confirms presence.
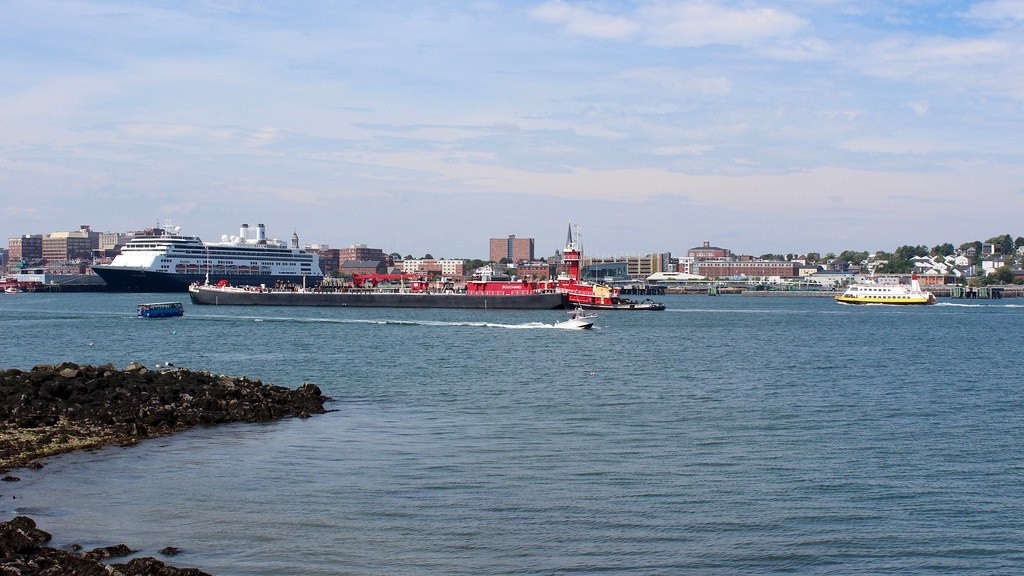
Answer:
[91, 223, 324, 292]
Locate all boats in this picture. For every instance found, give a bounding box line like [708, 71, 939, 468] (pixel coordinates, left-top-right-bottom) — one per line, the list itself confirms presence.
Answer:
[187, 282, 570, 309]
[554, 317, 594, 329]
[835, 273, 936, 305]
[569, 298, 665, 311]
[138, 301, 183, 317]
